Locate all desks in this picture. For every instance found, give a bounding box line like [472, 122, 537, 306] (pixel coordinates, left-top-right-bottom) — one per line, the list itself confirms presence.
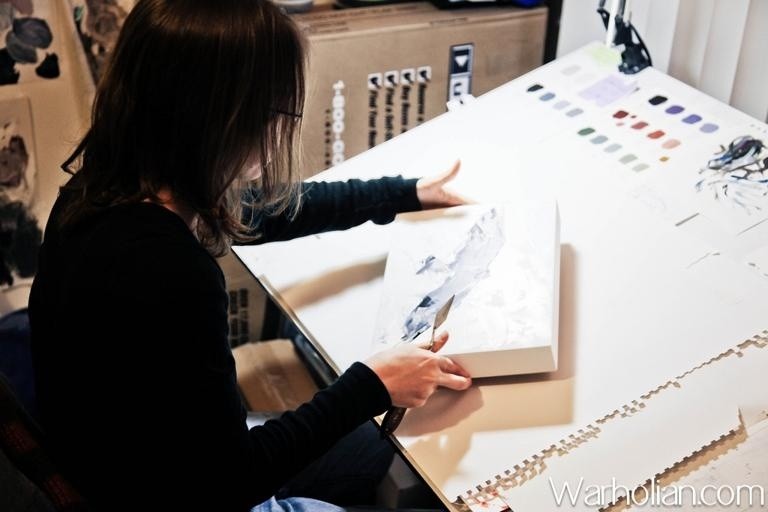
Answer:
[230, 41, 767, 510]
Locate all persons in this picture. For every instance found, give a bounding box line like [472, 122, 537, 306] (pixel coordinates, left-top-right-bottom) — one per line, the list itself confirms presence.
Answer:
[28, 0, 472, 512]
